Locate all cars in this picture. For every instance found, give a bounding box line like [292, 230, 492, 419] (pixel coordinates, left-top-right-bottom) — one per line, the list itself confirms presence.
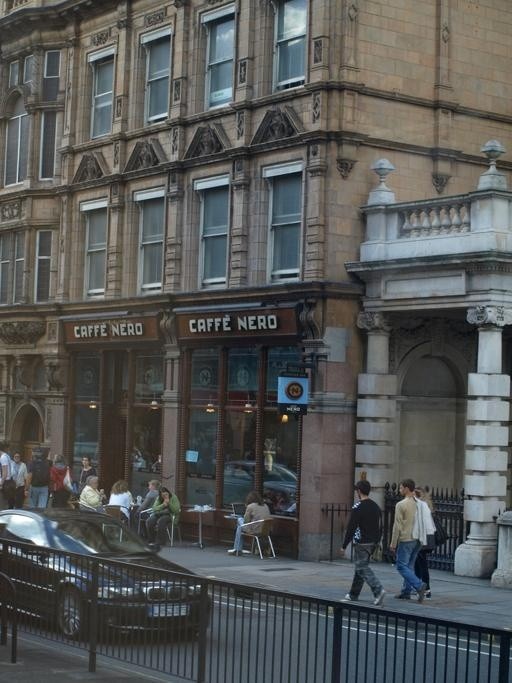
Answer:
[2, 505, 213, 646]
[222, 461, 298, 513]
[71, 441, 162, 473]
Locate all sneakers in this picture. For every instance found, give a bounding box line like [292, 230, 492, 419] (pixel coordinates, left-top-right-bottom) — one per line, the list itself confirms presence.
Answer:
[418, 583, 427, 602]
[423, 590, 431, 600]
[345, 593, 357, 600]
[374, 590, 385, 604]
[395, 593, 411, 600]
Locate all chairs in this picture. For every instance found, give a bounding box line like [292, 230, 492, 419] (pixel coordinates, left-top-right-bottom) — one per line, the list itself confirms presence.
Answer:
[75, 500, 181, 548]
[235, 518, 277, 560]
[232, 503, 246, 514]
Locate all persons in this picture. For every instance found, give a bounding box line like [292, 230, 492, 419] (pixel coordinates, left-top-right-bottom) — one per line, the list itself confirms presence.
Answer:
[0, 442, 185, 555]
[273, 488, 296, 511]
[228, 489, 272, 558]
[408, 485, 433, 601]
[340, 479, 386, 606]
[389, 478, 427, 602]
[263, 487, 277, 514]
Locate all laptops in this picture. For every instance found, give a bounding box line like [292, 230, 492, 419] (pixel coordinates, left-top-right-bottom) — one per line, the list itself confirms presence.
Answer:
[232, 503, 247, 517]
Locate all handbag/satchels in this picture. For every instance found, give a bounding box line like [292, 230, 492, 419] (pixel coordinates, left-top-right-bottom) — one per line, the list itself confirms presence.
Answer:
[432, 515, 449, 545]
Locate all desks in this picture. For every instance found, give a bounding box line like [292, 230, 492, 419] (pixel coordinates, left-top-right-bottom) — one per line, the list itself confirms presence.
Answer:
[225, 513, 259, 554]
[186, 507, 218, 549]
